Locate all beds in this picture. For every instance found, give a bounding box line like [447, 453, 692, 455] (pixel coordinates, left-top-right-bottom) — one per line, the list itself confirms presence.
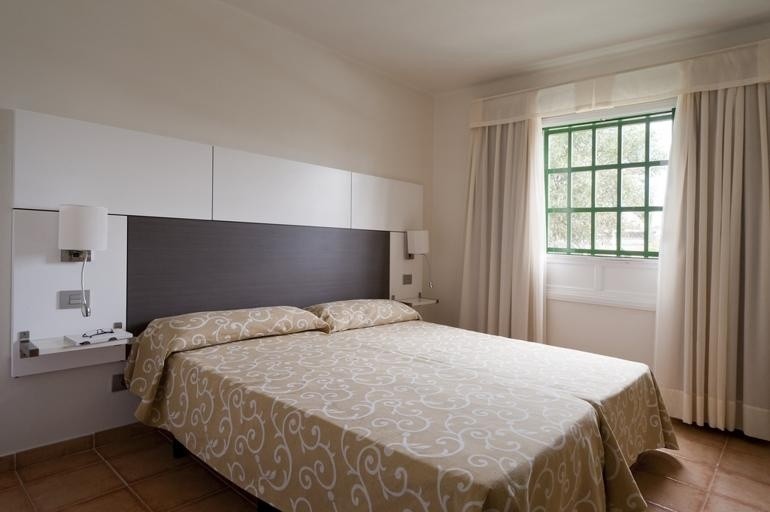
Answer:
[127, 216, 678, 512]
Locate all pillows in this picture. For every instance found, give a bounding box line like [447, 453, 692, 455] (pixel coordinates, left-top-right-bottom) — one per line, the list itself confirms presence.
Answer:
[306, 299, 419, 331]
[139, 305, 329, 359]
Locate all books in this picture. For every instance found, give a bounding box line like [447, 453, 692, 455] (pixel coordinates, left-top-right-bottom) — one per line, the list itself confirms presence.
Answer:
[63, 327, 134, 346]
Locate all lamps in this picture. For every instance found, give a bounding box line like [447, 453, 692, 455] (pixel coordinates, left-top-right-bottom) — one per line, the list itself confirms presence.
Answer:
[55, 202, 108, 317]
[405, 230, 434, 288]
